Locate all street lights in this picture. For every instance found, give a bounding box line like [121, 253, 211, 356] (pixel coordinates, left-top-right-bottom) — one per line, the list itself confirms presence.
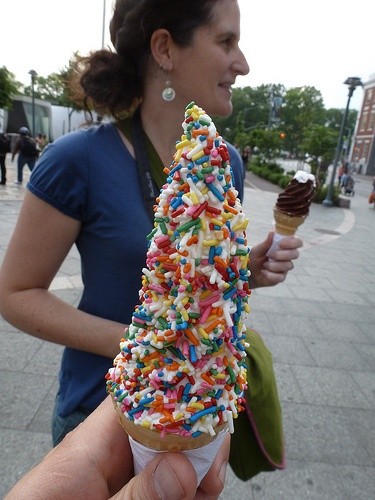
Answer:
[322, 77, 364, 207]
[28, 69, 38, 138]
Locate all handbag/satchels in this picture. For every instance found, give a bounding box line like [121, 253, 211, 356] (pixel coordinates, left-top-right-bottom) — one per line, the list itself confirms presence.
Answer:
[229, 326, 286, 482]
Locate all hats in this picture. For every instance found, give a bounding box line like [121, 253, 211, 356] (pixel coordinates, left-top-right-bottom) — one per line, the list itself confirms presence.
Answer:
[17, 127, 29, 135]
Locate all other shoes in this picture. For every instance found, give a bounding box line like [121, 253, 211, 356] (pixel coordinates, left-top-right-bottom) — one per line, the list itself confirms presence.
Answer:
[0, 178, 6, 184]
[14, 180, 22, 185]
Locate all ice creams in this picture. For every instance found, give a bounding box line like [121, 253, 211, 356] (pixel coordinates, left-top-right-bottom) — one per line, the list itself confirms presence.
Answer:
[104, 101, 250, 492]
[267, 171, 317, 262]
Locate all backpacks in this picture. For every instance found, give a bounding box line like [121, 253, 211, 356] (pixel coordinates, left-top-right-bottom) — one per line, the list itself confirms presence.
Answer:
[20, 137, 38, 159]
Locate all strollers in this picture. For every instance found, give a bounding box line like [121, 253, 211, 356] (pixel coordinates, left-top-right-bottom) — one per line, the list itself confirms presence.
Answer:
[340, 172, 356, 197]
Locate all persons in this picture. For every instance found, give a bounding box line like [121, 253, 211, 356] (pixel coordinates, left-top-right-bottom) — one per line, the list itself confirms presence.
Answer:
[0, 0, 304, 444]
[234, 139, 252, 179]
[0, 122, 50, 183]
[339, 157, 349, 189]
[3, 392, 231, 500]
